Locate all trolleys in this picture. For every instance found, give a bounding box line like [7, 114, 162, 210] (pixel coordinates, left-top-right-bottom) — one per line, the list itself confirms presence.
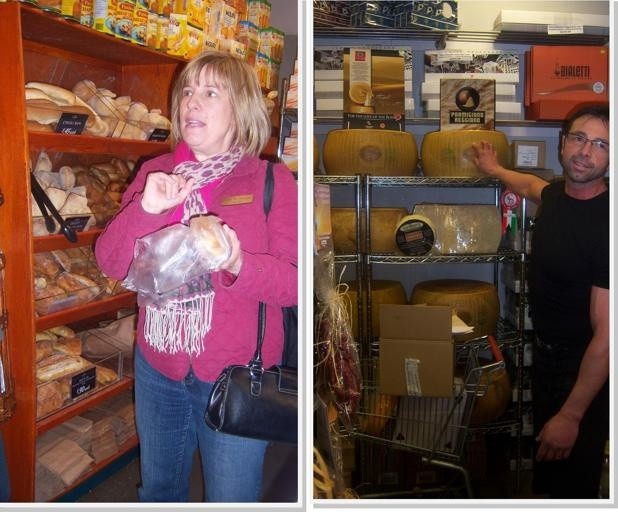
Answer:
[338, 336, 506, 498]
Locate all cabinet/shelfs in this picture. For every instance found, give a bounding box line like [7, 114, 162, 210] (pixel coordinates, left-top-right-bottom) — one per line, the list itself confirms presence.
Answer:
[313, 33, 610, 184]
[365, 174, 528, 498]
[0, 1, 279, 502]
[314, 175, 364, 437]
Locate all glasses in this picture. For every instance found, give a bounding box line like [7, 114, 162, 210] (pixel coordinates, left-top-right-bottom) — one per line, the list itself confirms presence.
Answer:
[565, 131, 608, 153]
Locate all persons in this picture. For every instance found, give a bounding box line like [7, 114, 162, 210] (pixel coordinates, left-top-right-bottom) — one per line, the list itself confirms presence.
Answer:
[94, 51, 298, 502]
[464, 101, 608, 499]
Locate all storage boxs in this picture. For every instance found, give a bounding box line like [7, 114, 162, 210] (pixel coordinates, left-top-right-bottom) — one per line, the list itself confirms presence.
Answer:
[313, 0, 350, 27]
[377, 303, 455, 397]
[530, 45, 608, 104]
[350, 2, 394, 28]
[510, 140, 545, 170]
[524, 101, 609, 120]
[493, 8, 609, 44]
[393, 1, 458, 31]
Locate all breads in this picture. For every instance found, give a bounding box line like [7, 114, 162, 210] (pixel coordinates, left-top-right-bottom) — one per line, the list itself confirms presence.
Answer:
[74, 78, 170, 141]
[25, 78, 107, 138]
[36, 325, 115, 418]
[33, 247, 131, 314]
[27, 150, 142, 232]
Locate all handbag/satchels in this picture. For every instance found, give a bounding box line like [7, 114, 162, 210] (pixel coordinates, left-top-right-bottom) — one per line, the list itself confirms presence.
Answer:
[206, 361, 297, 444]
